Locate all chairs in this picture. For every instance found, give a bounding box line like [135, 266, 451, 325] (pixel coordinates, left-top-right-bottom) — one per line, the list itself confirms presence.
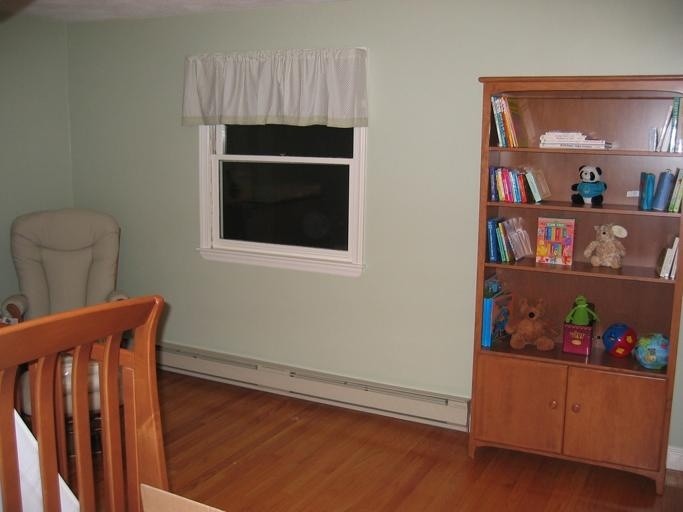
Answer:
[0, 208, 129, 460]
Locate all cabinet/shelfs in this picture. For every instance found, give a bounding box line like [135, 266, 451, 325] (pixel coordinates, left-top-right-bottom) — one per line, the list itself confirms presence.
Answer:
[465, 74, 683, 498]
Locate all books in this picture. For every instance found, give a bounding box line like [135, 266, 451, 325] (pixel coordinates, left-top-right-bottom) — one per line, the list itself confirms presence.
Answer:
[487, 95, 612, 265]
[482, 290, 511, 349]
[638, 94, 683, 280]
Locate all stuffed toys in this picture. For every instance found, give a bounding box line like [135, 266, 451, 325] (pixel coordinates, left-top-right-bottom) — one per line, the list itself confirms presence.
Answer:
[583, 224, 627, 268]
[503, 295, 558, 353]
[566, 293, 601, 325]
